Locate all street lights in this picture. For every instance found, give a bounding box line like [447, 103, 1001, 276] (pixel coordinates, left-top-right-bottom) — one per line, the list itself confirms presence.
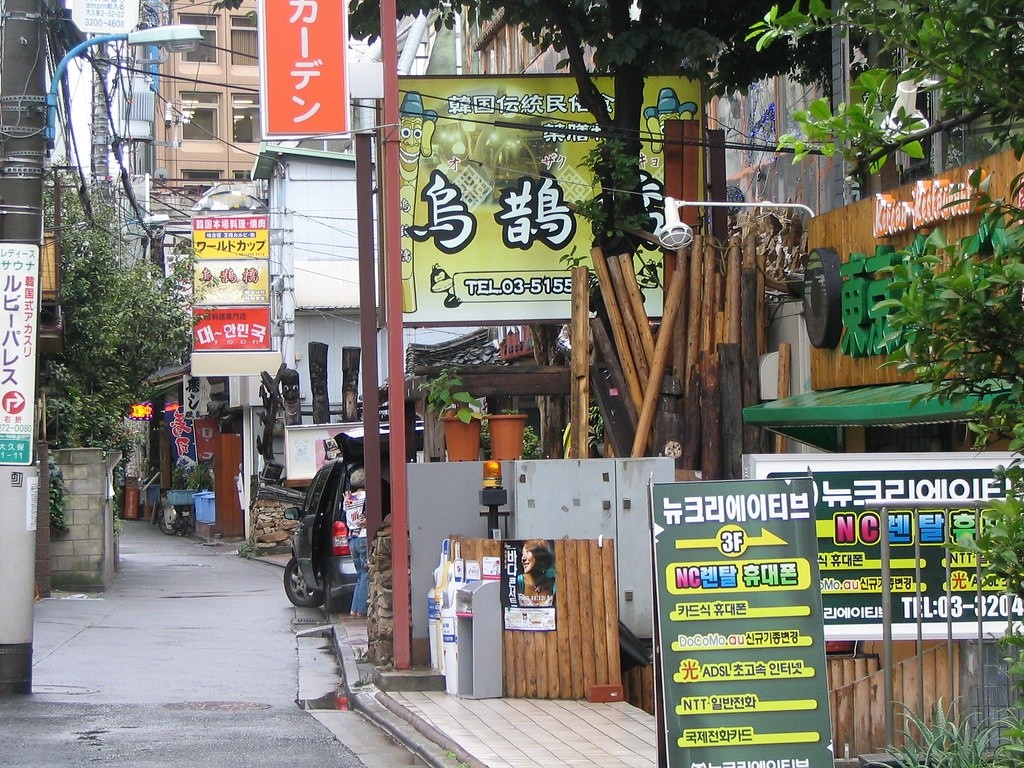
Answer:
[0, 24, 205, 694]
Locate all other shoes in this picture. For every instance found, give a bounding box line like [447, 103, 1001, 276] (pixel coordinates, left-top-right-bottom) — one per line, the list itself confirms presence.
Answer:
[349, 614, 367, 618]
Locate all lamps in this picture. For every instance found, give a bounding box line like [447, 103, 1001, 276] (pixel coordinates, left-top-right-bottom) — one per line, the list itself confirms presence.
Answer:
[482, 460, 503, 488]
[658, 197, 693, 250]
[878, 69, 939, 143]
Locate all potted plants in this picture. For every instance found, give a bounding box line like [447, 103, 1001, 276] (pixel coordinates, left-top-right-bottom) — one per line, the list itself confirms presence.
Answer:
[417, 367, 484, 463]
[485, 408, 529, 460]
[587, 398, 605, 458]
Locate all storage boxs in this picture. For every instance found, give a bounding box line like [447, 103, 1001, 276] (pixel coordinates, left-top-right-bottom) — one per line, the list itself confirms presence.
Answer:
[150, 484, 160, 504]
[192, 491, 215, 522]
[166, 490, 197, 506]
[201, 494, 215, 524]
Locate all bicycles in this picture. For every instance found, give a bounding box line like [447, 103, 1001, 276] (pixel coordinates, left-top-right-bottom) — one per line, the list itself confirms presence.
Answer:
[159, 490, 189, 536]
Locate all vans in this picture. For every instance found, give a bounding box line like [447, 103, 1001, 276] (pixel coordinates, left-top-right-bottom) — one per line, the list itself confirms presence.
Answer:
[284, 420, 424, 614]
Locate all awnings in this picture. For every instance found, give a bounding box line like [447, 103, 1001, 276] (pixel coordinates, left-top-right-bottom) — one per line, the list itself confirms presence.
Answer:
[743, 376, 1024, 452]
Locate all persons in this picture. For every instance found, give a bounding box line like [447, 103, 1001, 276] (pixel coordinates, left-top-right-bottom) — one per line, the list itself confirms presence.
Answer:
[517, 539, 556, 606]
[341, 467, 369, 618]
